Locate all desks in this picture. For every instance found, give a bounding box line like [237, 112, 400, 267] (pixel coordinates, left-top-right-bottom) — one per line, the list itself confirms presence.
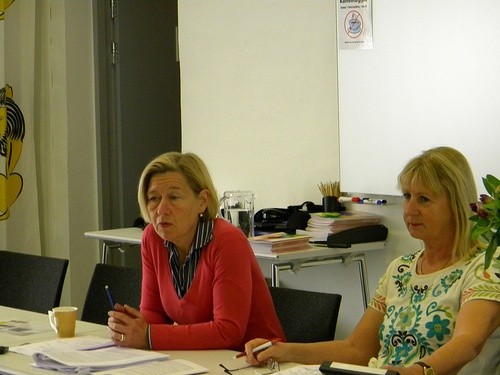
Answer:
[83, 227, 385, 312]
[0, 306, 305, 375]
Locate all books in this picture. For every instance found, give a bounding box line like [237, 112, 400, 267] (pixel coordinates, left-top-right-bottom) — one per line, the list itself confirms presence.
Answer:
[247, 212, 382, 256]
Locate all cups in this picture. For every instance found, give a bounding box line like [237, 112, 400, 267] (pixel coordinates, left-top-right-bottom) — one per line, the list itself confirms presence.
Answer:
[323, 196, 338, 212]
[218, 191, 255, 238]
[48, 306, 78, 337]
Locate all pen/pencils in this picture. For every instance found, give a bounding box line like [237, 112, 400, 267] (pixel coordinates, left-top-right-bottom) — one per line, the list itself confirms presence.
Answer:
[105, 286, 116, 311]
[232, 338, 283, 359]
[317, 180, 340, 196]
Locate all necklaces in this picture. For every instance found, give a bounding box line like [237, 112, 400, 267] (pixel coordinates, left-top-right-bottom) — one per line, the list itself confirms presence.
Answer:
[420, 256, 452, 274]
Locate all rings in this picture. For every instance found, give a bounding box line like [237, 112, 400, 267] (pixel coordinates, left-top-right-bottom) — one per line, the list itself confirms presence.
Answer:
[120, 334, 125, 341]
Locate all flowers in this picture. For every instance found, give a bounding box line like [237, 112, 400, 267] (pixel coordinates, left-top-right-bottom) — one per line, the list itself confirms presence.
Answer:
[467, 174, 500, 271]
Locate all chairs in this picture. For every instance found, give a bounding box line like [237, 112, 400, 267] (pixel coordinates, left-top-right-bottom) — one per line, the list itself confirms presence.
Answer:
[80, 264, 143, 328]
[268, 286, 343, 344]
[0, 250, 70, 315]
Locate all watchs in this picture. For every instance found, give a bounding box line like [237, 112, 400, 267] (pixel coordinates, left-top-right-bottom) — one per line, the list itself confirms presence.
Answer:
[413, 361, 435, 375]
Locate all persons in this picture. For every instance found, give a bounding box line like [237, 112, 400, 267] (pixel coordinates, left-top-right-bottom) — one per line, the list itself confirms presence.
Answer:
[245, 147, 500, 375]
[105, 152, 286, 352]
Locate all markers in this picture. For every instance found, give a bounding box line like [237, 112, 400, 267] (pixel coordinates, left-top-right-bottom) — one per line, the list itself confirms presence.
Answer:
[361, 196, 388, 205]
[337, 196, 359, 204]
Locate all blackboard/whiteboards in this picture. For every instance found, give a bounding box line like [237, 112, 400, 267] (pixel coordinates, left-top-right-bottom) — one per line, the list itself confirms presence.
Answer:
[333, 1, 500, 210]
